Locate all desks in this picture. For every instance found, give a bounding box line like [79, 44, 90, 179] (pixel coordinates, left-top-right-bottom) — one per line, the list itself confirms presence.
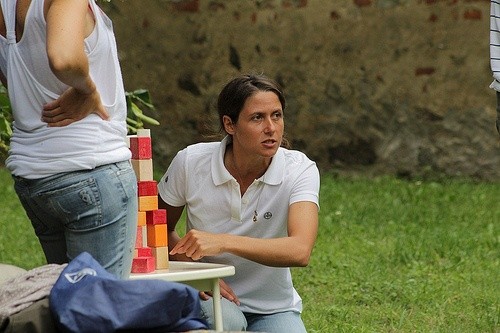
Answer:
[128, 261, 235, 332]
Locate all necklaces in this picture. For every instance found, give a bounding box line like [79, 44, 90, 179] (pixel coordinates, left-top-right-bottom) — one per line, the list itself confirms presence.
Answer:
[230, 156, 268, 221]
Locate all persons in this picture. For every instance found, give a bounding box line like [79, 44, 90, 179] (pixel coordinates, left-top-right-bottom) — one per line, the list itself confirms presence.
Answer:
[158, 74, 320, 333]
[0, 0, 136, 278]
